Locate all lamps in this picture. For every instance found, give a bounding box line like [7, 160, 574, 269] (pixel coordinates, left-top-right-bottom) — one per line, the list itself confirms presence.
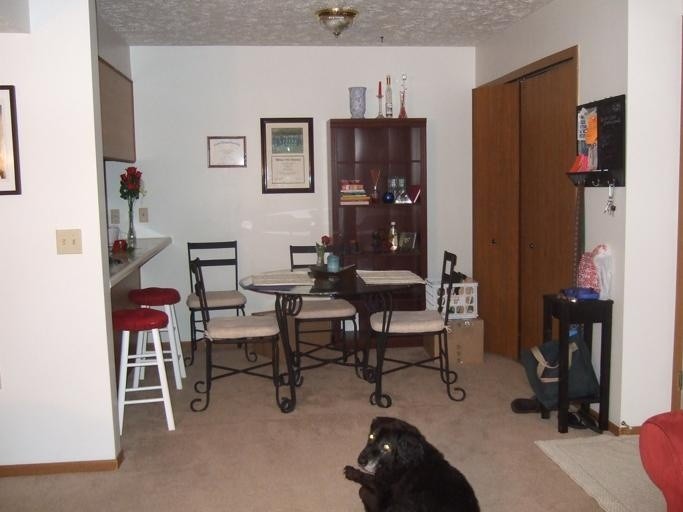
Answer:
[313, 5, 358, 37]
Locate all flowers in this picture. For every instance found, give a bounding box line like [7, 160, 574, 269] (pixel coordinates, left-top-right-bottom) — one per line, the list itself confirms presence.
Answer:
[315, 236, 329, 263]
[119, 164, 146, 236]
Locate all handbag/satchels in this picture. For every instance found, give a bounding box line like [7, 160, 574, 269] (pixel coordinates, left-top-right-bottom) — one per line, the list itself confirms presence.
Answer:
[520, 332, 600, 410]
[561, 287, 599, 301]
[576, 253, 600, 293]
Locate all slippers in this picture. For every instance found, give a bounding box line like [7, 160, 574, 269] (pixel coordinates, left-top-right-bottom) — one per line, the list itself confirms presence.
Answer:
[557, 411, 588, 429]
[581, 414, 603, 434]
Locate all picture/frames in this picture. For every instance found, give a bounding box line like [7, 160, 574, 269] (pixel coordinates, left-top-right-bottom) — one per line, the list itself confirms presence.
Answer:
[0, 84, 23, 195]
[258, 116, 317, 195]
[206, 134, 249, 169]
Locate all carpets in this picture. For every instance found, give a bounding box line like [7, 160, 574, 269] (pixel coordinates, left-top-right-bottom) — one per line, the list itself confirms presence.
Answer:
[534, 426, 669, 512]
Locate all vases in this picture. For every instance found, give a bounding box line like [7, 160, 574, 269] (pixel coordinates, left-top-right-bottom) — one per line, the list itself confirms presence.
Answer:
[126, 207, 135, 246]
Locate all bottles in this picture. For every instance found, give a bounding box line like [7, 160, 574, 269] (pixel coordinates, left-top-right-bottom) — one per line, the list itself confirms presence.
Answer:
[388, 220, 399, 252]
[385, 75, 393, 118]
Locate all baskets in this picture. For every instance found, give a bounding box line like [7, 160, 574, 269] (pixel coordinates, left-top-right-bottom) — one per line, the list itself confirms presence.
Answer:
[425, 273, 478, 320]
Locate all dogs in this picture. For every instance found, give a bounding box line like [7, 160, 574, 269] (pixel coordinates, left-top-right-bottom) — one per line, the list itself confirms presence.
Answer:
[342, 416, 481, 512]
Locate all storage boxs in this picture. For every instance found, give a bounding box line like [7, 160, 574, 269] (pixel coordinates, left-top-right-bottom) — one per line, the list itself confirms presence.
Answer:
[422, 317, 483, 365]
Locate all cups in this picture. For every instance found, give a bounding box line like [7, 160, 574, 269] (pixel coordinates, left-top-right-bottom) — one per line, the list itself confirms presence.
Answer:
[387, 175, 407, 193]
[326, 254, 340, 274]
[108, 227, 119, 247]
[348, 240, 360, 254]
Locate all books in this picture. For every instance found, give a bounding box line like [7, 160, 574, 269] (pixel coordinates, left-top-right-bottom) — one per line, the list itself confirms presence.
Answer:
[339, 188, 370, 206]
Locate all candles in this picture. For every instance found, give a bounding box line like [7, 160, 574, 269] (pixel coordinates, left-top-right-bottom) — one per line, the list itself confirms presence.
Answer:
[377, 80, 381, 95]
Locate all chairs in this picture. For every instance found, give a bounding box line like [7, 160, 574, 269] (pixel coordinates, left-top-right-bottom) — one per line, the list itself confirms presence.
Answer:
[188, 257, 294, 414]
[283, 244, 364, 386]
[184, 241, 258, 367]
[360, 250, 465, 409]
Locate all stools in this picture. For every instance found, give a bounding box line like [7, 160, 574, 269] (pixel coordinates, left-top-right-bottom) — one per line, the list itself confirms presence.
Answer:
[111, 308, 177, 437]
[127, 284, 191, 391]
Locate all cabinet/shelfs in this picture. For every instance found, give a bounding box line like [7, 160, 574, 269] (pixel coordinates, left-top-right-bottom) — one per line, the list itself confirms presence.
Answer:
[538, 291, 612, 433]
[325, 116, 429, 349]
